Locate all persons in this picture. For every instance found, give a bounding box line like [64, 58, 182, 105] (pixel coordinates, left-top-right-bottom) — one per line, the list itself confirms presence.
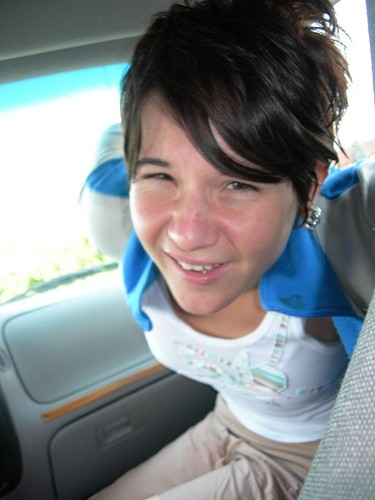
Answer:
[77, 0, 375, 500]
